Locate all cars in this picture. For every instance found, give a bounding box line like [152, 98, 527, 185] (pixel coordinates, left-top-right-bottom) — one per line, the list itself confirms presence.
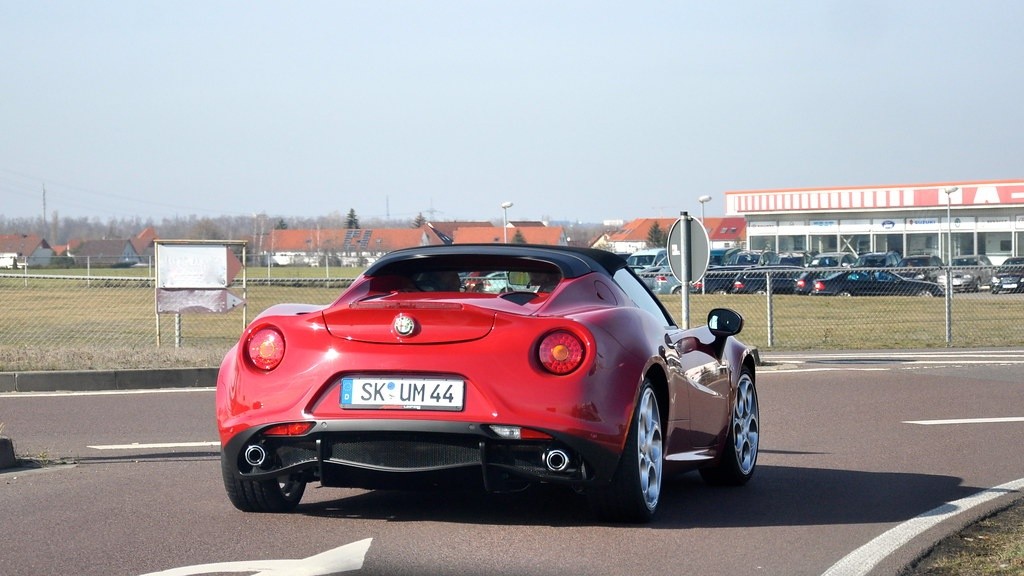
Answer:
[989, 256, 1024, 294]
[625, 246, 684, 297]
[893, 253, 943, 282]
[458, 270, 541, 293]
[810, 267, 946, 299]
[704, 248, 856, 296]
[938, 255, 996, 294]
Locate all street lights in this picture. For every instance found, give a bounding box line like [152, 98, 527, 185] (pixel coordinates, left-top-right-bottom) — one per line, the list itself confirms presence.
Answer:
[501, 202, 513, 243]
[944, 183, 959, 301]
[699, 194, 711, 295]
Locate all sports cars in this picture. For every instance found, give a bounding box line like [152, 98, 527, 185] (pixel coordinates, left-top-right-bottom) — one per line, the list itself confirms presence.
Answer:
[214, 242, 761, 526]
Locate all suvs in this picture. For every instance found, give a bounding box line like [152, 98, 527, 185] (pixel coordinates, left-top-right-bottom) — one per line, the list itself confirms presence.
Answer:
[847, 251, 902, 280]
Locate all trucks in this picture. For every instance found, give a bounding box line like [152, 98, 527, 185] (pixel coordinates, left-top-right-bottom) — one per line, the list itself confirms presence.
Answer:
[0, 252, 28, 269]
